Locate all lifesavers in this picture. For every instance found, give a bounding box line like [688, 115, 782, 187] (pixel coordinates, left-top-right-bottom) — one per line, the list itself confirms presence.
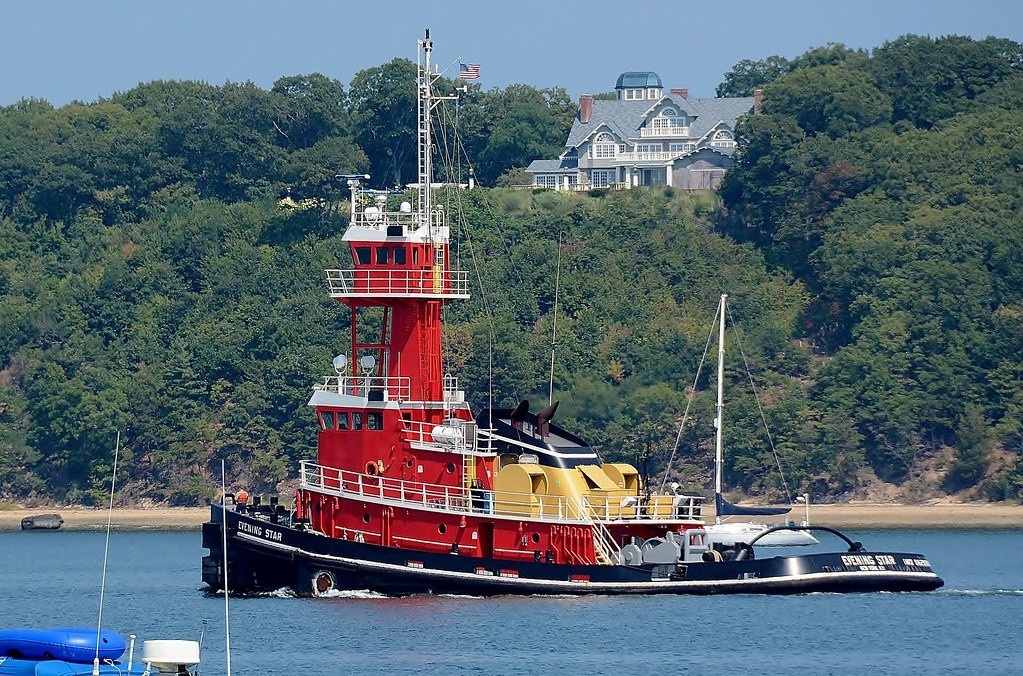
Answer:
[365, 461, 378, 479]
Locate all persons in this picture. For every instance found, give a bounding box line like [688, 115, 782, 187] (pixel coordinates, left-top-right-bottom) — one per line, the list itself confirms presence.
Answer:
[234, 485, 249, 515]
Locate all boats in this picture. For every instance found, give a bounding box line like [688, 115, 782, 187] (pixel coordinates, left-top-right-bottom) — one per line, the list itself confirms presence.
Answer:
[197, 24, 949, 594]
[0, 427, 236, 676]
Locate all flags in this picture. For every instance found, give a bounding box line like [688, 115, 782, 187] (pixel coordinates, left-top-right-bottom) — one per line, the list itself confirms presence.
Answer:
[460, 64, 480, 79]
